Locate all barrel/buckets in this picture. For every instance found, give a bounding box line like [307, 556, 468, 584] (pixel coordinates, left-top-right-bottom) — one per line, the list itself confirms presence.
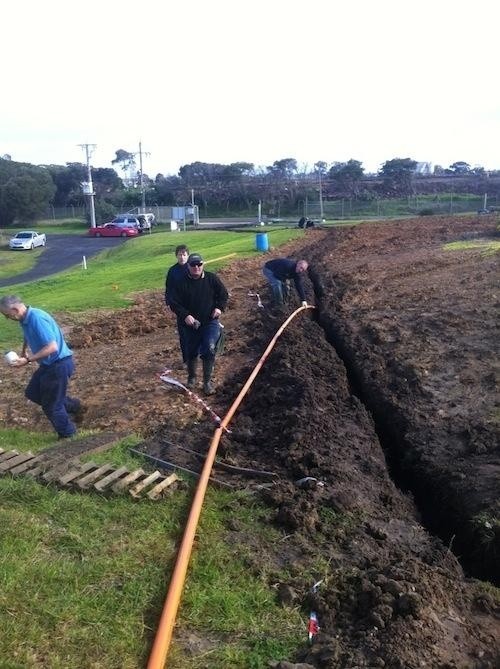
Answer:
[256, 233, 269, 252]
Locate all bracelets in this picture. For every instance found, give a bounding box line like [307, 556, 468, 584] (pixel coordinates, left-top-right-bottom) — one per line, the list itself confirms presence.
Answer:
[26, 357, 32, 365]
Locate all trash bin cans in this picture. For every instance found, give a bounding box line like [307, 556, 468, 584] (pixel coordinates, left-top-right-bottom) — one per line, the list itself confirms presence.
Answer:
[256, 233, 268, 251]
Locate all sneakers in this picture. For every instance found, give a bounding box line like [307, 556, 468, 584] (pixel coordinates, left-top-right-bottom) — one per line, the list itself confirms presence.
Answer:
[70, 398, 88, 423]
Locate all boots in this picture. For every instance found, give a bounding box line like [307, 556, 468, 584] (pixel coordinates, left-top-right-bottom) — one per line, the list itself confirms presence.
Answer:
[186, 354, 198, 389]
[200, 354, 216, 395]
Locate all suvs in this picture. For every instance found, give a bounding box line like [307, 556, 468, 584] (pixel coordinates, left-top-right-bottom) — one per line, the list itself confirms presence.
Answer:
[103, 217, 149, 233]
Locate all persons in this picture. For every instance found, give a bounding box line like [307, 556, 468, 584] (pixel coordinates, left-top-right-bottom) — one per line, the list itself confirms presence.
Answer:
[0, 296, 88, 442]
[262, 258, 309, 311]
[165, 245, 204, 363]
[169, 254, 228, 396]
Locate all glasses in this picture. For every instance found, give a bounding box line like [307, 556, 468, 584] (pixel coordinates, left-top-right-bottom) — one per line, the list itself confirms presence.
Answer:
[189, 263, 202, 267]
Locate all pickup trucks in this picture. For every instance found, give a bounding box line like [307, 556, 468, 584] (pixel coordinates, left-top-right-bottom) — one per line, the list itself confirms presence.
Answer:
[9, 231, 47, 251]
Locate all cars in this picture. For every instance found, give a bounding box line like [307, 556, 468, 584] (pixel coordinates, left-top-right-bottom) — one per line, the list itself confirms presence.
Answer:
[87, 224, 138, 237]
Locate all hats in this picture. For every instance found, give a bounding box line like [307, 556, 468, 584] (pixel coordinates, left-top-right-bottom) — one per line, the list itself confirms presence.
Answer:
[186, 253, 202, 264]
